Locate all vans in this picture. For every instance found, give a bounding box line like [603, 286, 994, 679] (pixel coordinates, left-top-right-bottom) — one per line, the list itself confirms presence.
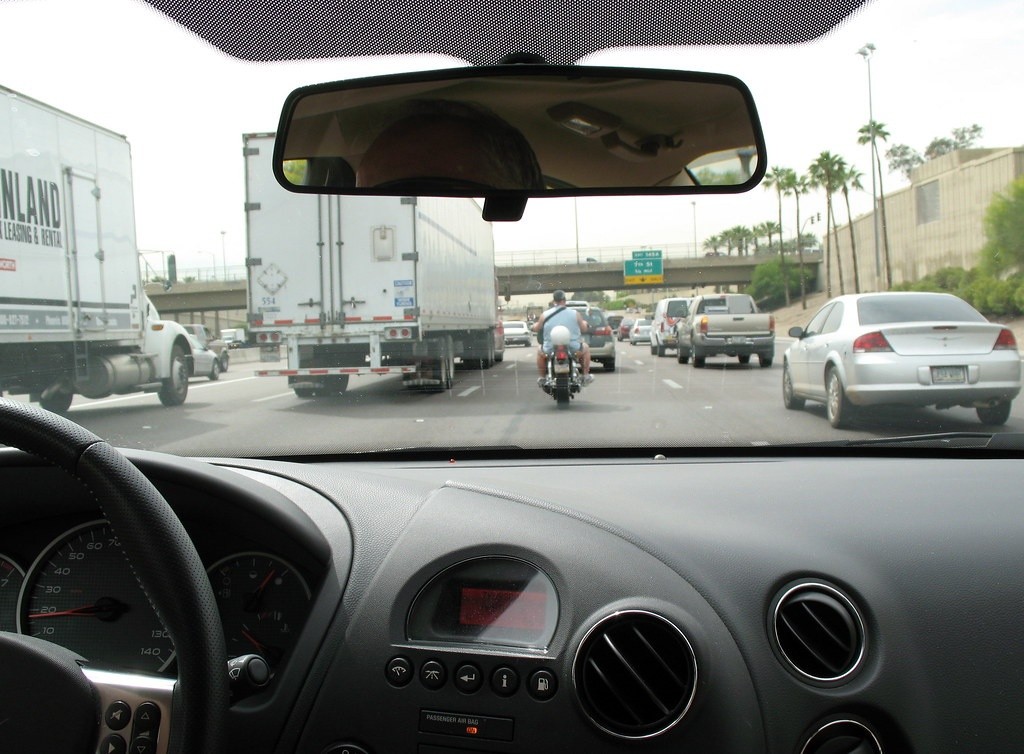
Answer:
[646, 298, 695, 358]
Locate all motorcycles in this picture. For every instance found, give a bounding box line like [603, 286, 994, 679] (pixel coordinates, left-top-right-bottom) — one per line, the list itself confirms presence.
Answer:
[528, 320, 594, 409]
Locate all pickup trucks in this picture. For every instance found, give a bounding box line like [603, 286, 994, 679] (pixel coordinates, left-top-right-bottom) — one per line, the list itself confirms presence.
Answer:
[673, 294, 775, 369]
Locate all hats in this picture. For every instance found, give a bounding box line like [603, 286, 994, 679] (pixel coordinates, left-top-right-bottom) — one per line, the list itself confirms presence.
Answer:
[554, 290, 566, 301]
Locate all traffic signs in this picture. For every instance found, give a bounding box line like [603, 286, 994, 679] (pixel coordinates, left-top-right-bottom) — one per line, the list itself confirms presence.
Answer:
[624, 258, 664, 285]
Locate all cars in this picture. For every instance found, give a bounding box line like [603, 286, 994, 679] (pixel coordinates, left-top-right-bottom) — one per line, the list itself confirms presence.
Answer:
[502, 320, 534, 347]
[227, 340, 243, 349]
[606, 316, 654, 346]
[180, 323, 232, 381]
[565, 305, 618, 372]
[781, 292, 1023, 429]
[703, 252, 726, 257]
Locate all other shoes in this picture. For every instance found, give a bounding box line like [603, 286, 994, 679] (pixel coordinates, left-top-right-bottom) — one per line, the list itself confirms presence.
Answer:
[537, 377, 545, 388]
[583, 374, 595, 387]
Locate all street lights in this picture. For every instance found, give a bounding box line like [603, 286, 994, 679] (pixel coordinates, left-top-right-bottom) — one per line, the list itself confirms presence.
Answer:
[691, 201, 697, 259]
[858, 42, 881, 294]
[220, 231, 227, 280]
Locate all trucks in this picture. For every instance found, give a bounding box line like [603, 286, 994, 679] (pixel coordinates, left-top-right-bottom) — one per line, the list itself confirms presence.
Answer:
[220, 328, 245, 345]
[0, 84, 196, 415]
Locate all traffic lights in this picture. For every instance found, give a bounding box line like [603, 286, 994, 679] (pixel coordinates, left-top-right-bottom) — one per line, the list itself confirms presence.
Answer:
[811, 216, 814, 224]
[817, 212, 821, 221]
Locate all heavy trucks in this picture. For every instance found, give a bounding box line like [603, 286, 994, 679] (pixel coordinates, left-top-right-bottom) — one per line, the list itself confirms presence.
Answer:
[244, 129, 510, 400]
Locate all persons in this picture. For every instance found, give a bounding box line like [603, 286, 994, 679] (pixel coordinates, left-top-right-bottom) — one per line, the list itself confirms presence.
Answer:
[533, 290, 594, 387]
[356, 99, 547, 188]
